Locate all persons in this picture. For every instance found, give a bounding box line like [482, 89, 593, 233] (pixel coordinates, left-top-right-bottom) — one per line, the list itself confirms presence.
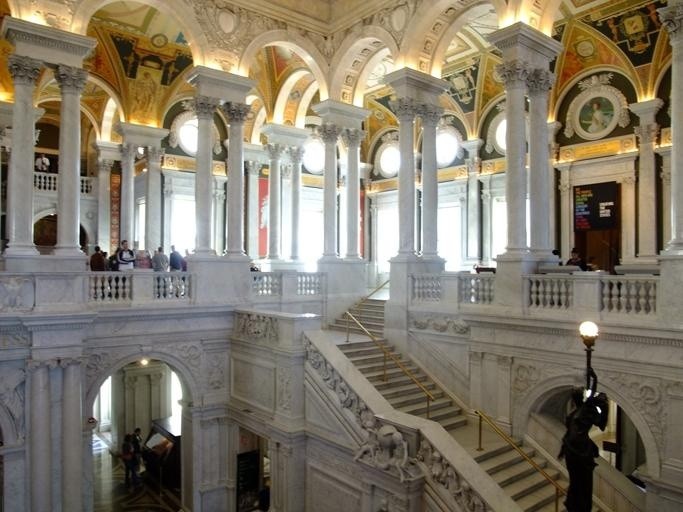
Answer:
[607, 17, 620, 42]
[553, 249, 562, 266]
[122, 434, 139, 487]
[34, 153, 50, 171]
[132, 428, 145, 471]
[464, 69, 475, 88]
[353, 420, 378, 463]
[566, 248, 587, 271]
[588, 102, 603, 133]
[90, 241, 182, 272]
[258, 479, 270, 512]
[392, 432, 413, 483]
[646, 4, 661, 30]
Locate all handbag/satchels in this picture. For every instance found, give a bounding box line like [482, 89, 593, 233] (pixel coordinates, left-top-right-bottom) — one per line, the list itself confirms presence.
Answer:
[121, 452, 131, 461]
